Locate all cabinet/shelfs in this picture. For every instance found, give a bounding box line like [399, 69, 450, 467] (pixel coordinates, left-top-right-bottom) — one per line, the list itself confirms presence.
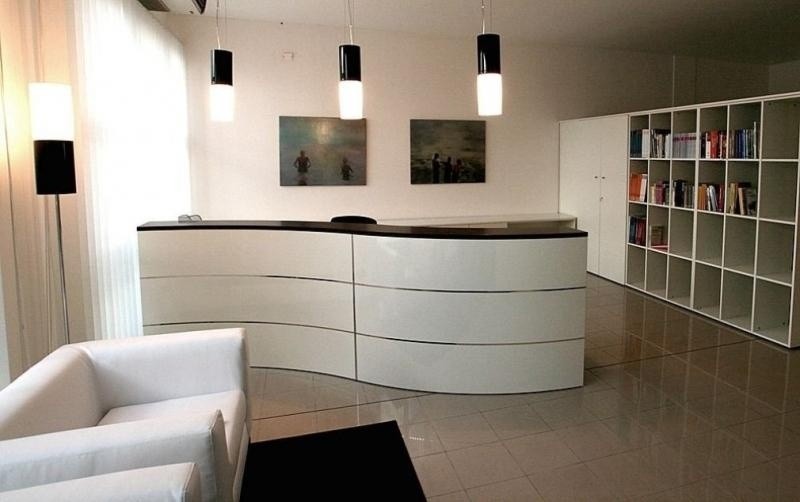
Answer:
[551, 89, 800, 351]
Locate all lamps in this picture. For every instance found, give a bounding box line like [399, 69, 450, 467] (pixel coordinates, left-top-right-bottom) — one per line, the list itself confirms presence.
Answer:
[336, 0, 364, 123]
[28, 78, 83, 347]
[206, 2, 235, 129]
[477, 2, 503, 121]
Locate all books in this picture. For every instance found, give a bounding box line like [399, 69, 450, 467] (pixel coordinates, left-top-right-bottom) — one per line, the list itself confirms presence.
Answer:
[629, 121, 759, 250]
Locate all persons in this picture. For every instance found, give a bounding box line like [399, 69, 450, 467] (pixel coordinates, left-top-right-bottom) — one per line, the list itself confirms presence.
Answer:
[440, 157, 452, 183]
[432, 152, 440, 183]
[453, 159, 461, 182]
[293, 150, 311, 172]
[338, 157, 355, 180]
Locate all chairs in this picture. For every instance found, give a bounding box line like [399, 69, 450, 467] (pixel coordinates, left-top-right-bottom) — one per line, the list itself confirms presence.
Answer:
[1, 326, 254, 502]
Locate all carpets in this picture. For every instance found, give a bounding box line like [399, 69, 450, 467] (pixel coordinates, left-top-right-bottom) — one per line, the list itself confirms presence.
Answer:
[246, 415, 430, 501]
